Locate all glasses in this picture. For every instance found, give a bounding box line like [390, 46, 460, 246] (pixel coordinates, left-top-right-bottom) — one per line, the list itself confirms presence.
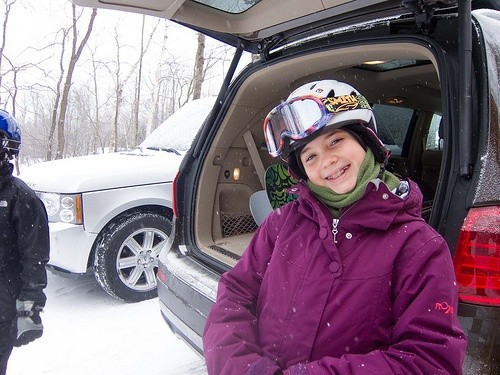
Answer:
[263, 95, 372, 158]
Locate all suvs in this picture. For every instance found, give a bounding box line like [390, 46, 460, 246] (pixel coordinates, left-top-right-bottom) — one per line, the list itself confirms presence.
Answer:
[17, 95, 222, 303]
[73, 0, 500, 375]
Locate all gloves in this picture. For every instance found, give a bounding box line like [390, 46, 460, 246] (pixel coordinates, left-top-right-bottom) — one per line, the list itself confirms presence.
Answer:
[10, 299, 44, 347]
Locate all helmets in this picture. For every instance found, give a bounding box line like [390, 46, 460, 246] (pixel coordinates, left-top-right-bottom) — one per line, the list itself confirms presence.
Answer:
[0, 109, 21, 166]
[280, 79, 391, 182]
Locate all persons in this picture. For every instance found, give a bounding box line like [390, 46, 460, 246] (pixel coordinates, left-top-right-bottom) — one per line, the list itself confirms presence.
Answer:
[202, 80, 465, 375]
[0, 108, 50, 375]
